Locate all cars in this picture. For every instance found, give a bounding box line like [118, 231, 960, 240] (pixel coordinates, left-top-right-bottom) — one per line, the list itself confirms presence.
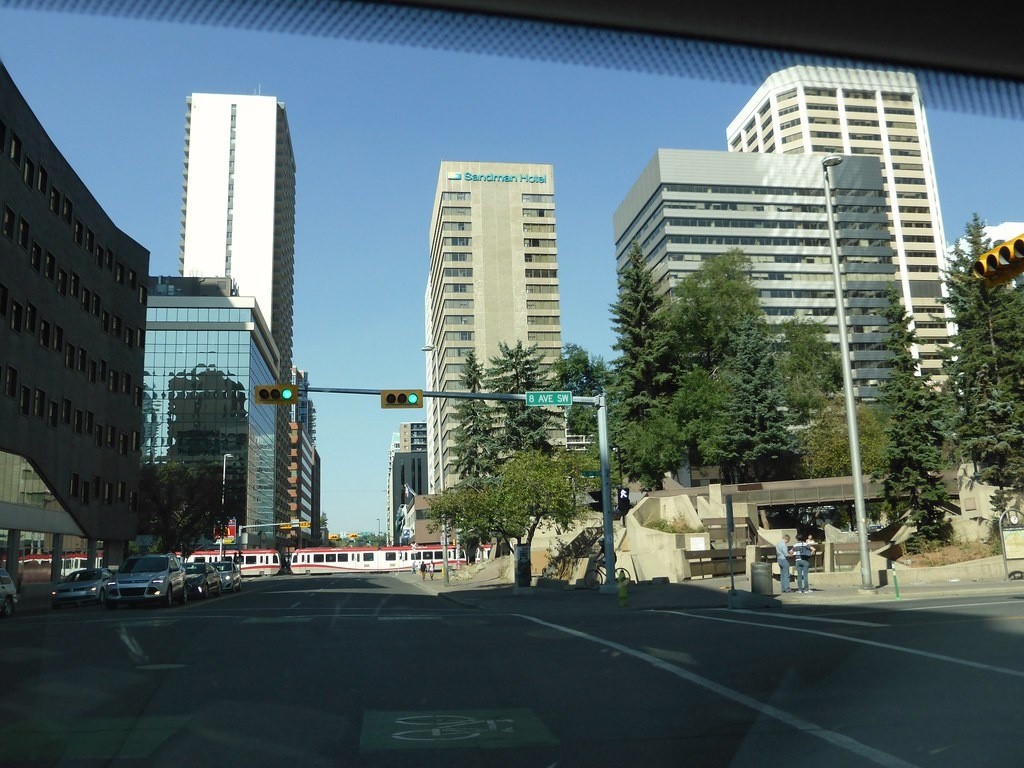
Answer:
[50, 568, 115, 610]
[184, 562, 223, 600]
[213, 562, 242, 594]
[867, 524, 883, 534]
[0, 568, 17, 617]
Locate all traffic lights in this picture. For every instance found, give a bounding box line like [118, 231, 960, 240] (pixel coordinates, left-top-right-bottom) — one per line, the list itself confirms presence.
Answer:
[380, 389, 424, 409]
[348, 533, 357, 539]
[971, 233, 1024, 291]
[589, 490, 602, 513]
[618, 487, 629, 511]
[328, 535, 339, 540]
[254, 384, 300, 404]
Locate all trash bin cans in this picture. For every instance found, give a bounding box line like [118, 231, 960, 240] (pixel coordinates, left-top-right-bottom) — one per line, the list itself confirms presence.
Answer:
[751, 562, 773, 596]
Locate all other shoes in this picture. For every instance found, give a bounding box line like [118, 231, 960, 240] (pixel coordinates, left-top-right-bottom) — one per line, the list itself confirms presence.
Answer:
[798, 591, 802, 593]
[805, 590, 812, 593]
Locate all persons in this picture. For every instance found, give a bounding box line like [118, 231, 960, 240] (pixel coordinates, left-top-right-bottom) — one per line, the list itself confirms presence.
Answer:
[429, 561, 435, 580]
[592, 538, 617, 576]
[789, 534, 816, 593]
[420, 562, 426, 580]
[412, 561, 421, 574]
[776, 535, 796, 593]
[806, 535, 815, 543]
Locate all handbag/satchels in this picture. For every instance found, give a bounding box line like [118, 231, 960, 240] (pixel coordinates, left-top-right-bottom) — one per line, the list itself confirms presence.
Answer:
[800, 542, 811, 558]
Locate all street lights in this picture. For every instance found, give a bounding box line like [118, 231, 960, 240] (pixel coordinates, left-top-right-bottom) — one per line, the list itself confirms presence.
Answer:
[820, 154, 874, 591]
[219, 454, 235, 562]
[377, 519, 380, 547]
[421, 344, 451, 588]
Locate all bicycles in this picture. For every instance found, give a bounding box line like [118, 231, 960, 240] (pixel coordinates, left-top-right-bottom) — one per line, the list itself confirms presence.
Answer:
[583, 559, 631, 590]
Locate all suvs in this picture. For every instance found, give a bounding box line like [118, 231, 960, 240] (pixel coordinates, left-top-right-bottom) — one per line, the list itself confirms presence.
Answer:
[104, 552, 186, 611]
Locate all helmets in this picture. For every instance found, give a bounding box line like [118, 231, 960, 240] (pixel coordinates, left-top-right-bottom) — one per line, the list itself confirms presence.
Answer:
[598, 538, 604, 542]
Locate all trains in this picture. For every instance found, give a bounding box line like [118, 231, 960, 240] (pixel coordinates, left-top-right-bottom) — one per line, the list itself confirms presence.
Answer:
[2, 544, 492, 577]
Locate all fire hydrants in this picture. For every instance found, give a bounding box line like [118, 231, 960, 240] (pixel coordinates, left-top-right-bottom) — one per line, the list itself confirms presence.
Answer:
[616, 569, 632, 608]
